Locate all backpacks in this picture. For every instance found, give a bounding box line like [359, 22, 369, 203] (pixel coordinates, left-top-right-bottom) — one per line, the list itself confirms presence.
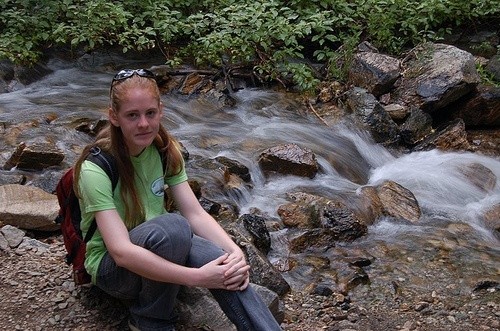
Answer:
[51, 166, 87, 286]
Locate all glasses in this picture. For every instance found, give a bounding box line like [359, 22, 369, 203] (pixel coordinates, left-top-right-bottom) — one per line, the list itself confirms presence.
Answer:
[110, 68, 158, 102]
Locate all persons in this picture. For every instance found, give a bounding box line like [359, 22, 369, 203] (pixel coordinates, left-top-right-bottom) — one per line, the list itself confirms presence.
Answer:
[70, 68, 282, 331]
